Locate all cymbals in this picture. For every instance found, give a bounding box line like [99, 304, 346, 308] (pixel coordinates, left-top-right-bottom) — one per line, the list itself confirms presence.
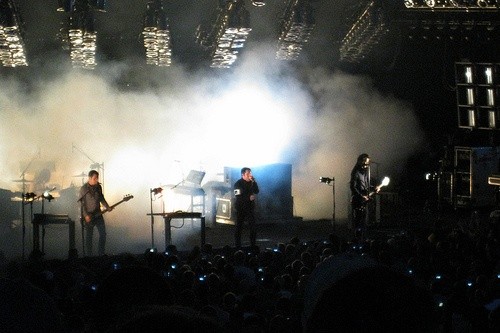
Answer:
[12, 180, 34, 182]
[72, 173, 88, 177]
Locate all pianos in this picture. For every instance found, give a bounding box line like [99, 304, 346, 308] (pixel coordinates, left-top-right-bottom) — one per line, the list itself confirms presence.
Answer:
[159, 184, 205, 231]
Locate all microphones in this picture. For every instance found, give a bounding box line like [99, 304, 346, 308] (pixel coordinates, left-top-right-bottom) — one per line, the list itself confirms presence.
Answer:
[48, 185, 57, 192]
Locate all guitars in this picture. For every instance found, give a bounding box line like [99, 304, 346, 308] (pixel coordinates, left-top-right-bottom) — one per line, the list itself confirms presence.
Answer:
[352, 176, 390, 212]
[79, 194, 134, 230]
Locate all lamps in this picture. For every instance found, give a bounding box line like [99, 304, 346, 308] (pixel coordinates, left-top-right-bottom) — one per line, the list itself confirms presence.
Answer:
[0, 0, 29, 67]
[55, 0, 107, 70]
[331, 0, 500, 65]
[142, 0, 173, 67]
[193, 0, 268, 72]
[268, 0, 315, 61]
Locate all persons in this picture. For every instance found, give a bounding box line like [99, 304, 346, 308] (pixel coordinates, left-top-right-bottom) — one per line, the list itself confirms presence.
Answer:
[34, 169, 49, 194]
[234, 168, 259, 247]
[350, 154, 380, 228]
[80, 170, 113, 256]
[0, 208, 500, 333]
